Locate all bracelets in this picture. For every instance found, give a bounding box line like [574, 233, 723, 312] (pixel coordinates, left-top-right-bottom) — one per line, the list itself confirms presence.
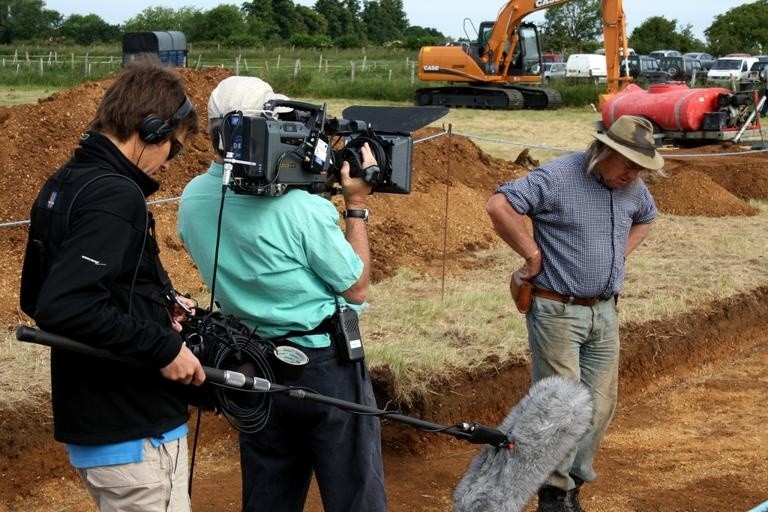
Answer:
[524, 246, 541, 261]
[341, 208, 372, 223]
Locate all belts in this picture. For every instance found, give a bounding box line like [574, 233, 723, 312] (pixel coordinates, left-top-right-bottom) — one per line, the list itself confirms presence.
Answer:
[531, 288, 601, 306]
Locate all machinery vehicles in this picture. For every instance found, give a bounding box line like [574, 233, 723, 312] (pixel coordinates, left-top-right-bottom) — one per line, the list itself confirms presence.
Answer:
[411, 0, 636, 111]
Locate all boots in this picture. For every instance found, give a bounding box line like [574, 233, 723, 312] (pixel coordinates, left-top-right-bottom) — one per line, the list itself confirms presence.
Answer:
[536, 487, 584, 512]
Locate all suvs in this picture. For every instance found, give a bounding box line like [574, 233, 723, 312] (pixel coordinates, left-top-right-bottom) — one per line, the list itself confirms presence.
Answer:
[525, 47, 768, 91]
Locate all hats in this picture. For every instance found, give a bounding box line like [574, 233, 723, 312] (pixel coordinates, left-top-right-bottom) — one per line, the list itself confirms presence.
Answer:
[591, 114, 664, 170]
[208, 75, 294, 120]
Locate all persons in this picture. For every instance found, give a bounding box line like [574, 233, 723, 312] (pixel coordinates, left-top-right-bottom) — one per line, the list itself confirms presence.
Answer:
[175, 74, 390, 512]
[17, 51, 208, 512]
[482, 113, 668, 512]
